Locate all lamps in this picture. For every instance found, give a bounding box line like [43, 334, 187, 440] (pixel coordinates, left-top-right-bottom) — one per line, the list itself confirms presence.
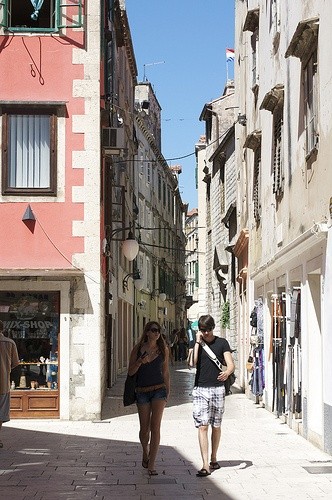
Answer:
[150, 288, 166, 301]
[122, 268, 143, 293]
[102, 222, 139, 261]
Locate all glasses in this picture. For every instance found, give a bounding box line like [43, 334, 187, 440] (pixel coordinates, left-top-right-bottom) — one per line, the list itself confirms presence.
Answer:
[147, 328, 161, 333]
[199, 326, 213, 333]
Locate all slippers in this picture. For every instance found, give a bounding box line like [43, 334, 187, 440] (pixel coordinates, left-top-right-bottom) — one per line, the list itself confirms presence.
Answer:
[210, 461, 220, 469]
[142, 458, 149, 468]
[196, 468, 211, 477]
[148, 469, 158, 475]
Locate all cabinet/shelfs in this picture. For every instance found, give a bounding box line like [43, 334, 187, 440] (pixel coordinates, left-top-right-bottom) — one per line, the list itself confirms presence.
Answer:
[9, 334, 60, 391]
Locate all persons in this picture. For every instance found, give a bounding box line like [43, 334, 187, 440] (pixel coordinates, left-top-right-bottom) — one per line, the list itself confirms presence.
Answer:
[169, 328, 195, 362]
[0, 320, 19, 448]
[128, 321, 169, 476]
[187, 314, 235, 477]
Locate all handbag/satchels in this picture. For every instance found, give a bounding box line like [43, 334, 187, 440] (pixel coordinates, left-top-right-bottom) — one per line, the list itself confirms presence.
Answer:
[123, 343, 140, 406]
[222, 365, 236, 386]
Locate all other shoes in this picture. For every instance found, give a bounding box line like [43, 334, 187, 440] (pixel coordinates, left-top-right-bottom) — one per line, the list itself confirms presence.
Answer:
[0, 440, 3, 448]
